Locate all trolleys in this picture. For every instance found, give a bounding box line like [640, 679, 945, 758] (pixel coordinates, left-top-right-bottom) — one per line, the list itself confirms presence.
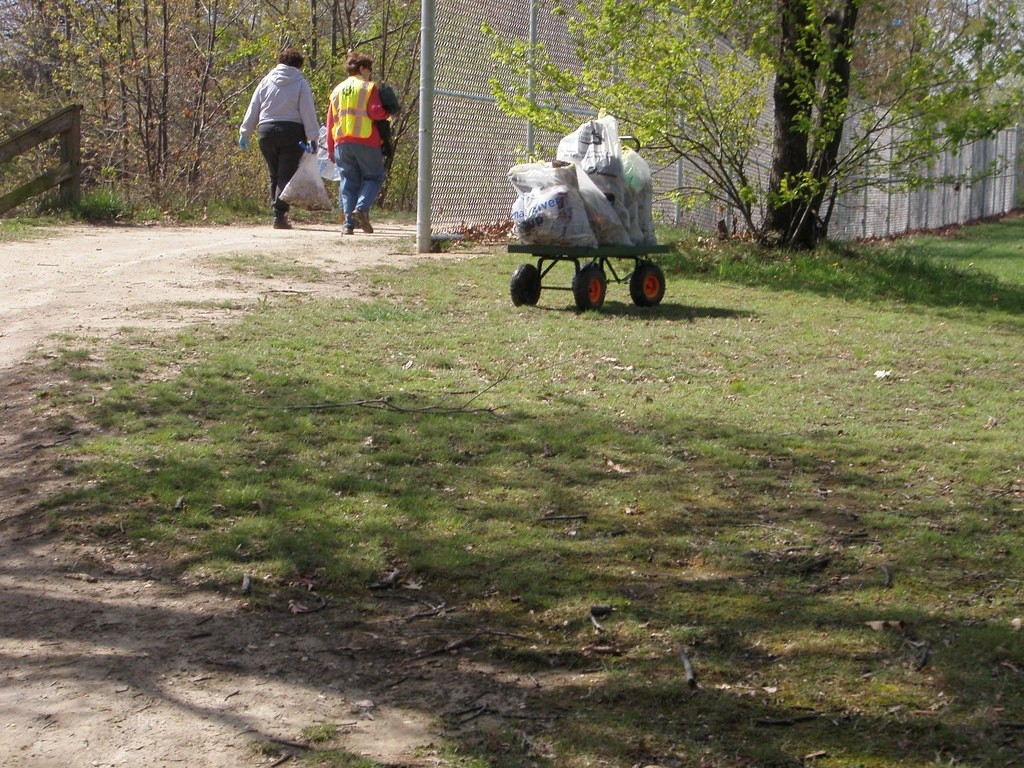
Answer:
[506, 136, 671, 313]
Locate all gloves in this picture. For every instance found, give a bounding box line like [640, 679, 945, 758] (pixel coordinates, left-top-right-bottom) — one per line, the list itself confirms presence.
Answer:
[310, 141, 317, 154]
[239, 136, 249, 151]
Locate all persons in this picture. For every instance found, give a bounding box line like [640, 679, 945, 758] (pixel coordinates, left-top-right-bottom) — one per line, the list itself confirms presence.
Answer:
[325, 51, 394, 235]
[238, 49, 320, 229]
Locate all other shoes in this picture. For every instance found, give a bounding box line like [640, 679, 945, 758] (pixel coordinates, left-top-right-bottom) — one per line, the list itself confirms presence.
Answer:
[273, 217, 292, 229]
[342, 227, 354, 235]
[352, 210, 373, 233]
